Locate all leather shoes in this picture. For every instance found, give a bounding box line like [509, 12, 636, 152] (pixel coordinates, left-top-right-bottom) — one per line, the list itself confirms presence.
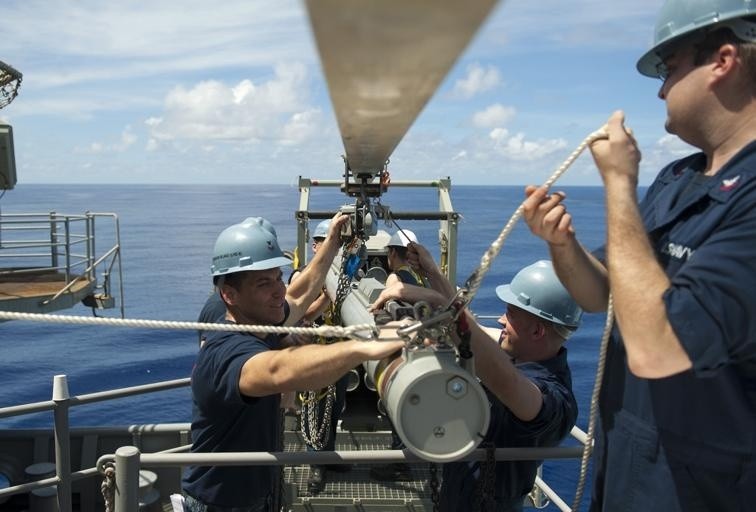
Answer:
[372, 462, 413, 481]
[308, 464, 352, 493]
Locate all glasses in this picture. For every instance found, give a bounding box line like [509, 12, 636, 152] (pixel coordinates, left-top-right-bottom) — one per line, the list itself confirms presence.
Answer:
[654, 60, 721, 80]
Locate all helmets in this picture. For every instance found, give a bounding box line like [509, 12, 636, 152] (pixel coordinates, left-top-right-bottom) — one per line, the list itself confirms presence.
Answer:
[311, 219, 342, 238]
[384, 229, 419, 249]
[494, 259, 585, 328]
[636, 0, 756, 76]
[211, 217, 294, 277]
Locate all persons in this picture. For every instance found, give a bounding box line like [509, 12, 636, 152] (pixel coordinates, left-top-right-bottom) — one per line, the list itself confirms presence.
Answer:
[370, 239, 585, 512]
[518, 0, 755, 512]
[177, 217, 436, 511]
[370, 229, 430, 486]
[193, 215, 332, 349]
[287, 219, 353, 496]
